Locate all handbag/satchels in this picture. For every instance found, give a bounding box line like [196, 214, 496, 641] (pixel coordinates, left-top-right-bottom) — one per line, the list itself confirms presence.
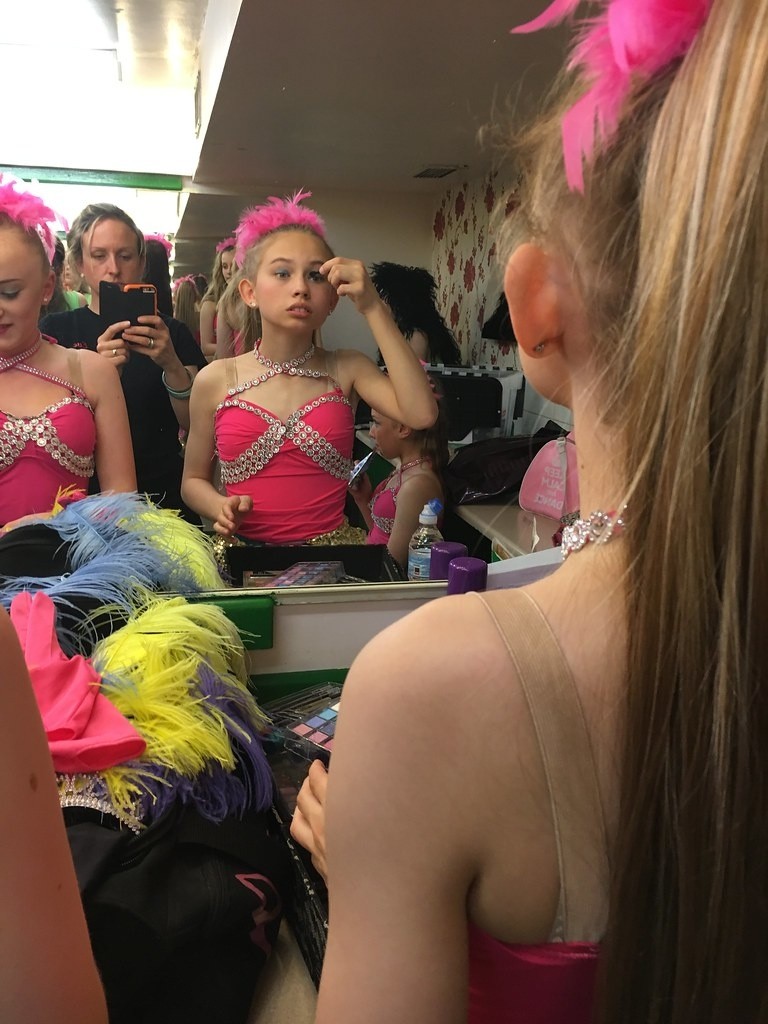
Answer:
[445, 420, 565, 507]
[519, 429, 581, 520]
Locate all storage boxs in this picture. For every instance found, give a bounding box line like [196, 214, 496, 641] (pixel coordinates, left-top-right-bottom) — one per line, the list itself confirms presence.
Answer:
[261, 561, 346, 587]
[284, 698, 340, 768]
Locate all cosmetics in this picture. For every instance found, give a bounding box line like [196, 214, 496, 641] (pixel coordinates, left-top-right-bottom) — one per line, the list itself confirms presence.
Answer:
[265, 560, 345, 586]
[285, 697, 340, 771]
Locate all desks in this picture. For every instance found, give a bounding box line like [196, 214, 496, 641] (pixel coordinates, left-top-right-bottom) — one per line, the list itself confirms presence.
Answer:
[455, 504, 561, 561]
[355, 430, 466, 467]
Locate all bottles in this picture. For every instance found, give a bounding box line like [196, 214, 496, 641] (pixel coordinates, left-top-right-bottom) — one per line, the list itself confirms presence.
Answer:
[407, 497, 444, 580]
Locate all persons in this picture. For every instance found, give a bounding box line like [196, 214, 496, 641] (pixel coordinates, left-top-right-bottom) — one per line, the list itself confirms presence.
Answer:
[0, 608, 109, 1024]
[370, 262, 460, 379]
[0, 174, 141, 653]
[347, 407, 443, 569]
[143, 236, 262, 362]
[181, 192, 438, 586]
[39, 203, 208, 532]
[291, 0, 767, 1024]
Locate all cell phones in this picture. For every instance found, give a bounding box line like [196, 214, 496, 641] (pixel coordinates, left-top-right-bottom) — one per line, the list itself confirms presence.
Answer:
[100, 280, 157, 346]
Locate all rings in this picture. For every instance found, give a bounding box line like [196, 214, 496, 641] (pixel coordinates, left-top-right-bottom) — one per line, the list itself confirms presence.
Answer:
[148, 337, 154, 349]
[113, 349, 116, 357]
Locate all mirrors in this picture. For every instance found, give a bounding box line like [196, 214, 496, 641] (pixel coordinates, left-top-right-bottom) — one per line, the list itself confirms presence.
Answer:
[0, 0, 606, 607]
